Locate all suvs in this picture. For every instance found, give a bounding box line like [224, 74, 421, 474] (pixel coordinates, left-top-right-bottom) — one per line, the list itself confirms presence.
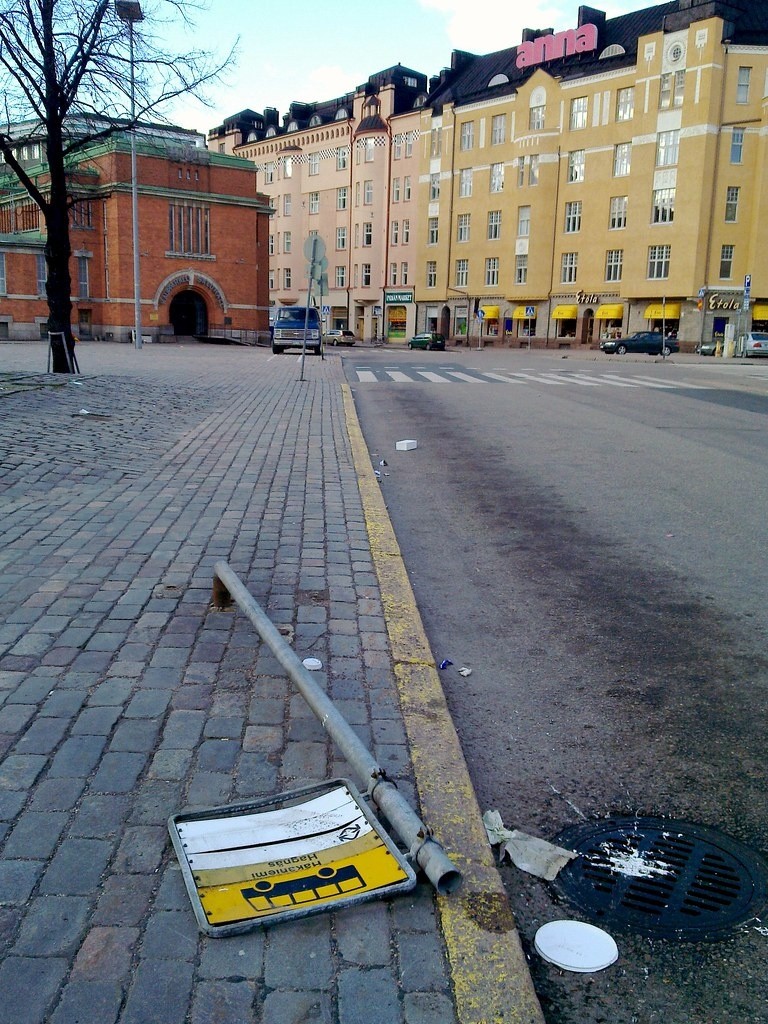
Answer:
[321, 328, 356, 346]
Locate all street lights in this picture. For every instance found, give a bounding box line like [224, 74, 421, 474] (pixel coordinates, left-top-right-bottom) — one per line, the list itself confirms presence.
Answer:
[112, 0, 148, 350]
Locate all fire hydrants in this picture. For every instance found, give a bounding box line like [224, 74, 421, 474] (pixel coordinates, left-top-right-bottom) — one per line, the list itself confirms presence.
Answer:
[714, 339, 722, 357]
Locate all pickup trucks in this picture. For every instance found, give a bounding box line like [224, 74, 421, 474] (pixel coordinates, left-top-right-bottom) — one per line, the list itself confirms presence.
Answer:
[270, 306, 326, 357]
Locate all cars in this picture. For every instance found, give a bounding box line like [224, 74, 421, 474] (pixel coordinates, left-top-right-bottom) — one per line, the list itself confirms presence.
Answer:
[408, 332, 445, 353]
[732, 332, 768, 359]
[695, 339, 725, 357]
[599, 330, 679, 357]
